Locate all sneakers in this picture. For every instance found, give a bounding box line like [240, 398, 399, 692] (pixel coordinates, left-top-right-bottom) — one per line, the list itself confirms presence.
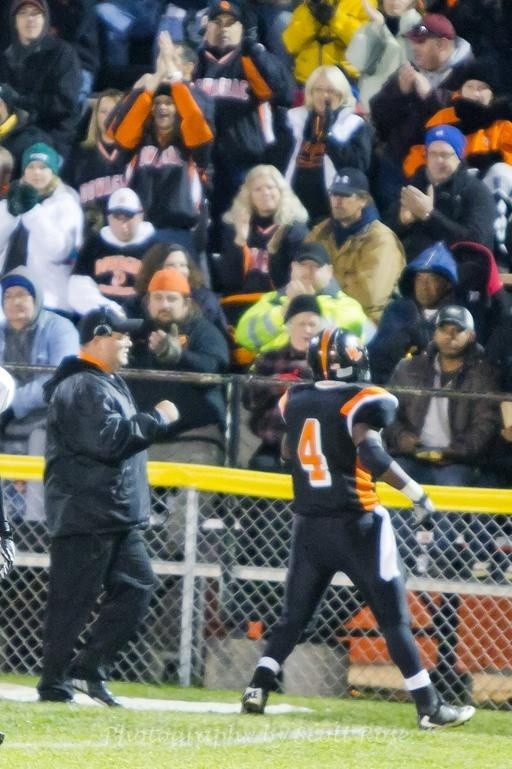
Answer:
[39, 694, 79, 704]
[240, 685, 267, 714]
[64, 677, 122, 709]
[416, 701, 477, 729]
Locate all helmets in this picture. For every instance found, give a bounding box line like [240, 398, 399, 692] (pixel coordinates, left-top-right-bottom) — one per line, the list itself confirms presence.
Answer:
[305, 325, 372, 383]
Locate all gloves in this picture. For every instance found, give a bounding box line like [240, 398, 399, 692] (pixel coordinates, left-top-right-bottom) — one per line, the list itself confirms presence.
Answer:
[412, 491, 434, 526]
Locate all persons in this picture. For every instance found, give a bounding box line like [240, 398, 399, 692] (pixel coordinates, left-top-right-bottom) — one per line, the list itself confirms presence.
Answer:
[370, 14, 474, 178]
[59, 90, 126, 239]
[384, 126, 495, 264]
[367, 241, 487, 385]
[403, 61, 512, 251]
[220, 165, 308, 295]
[0, 81, 13, 197]
[0, 363, 17, 745]
[130, 268, 229, 557]
[0, 142, 84, 315]
[122, 242, 229, 342]
[242, 329, 477, 728]
[0, 265, 80, 524]
[0, 0, 82, 155]
[36, 306, 178, 706]
[282, 0, 378, 102]
[381, 305, 502, 583]
[305, 169, 406, 326]
[234, 242, 366, 354]
[114, 31, 215, 265]
[67, 188, 169, 326]
[243, 294, 325, 473]
[347, 0, 422, 113]
[183, 0, 295, 292]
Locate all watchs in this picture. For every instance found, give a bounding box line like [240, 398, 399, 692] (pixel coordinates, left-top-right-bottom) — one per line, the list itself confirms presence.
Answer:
[168, 72, 183, 81]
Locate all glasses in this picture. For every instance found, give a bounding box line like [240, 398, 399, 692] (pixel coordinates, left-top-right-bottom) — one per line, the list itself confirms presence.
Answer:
[411, 23, 433, 38]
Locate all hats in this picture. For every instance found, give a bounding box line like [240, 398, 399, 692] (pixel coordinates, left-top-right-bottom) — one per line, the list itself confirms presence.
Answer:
[1, 274, 36, 306]
[283, 293, 321, 323]
[146, 268, 192, 294]
[423, 123, 467, 159]
[400, 11, 455, 42]
[328, 165, 369, 195]
[105, 186, 142, 211]
[153, 80, 171, 97]
[20, 141, 59, 176]
[295, 241, 330, 267]
[9, 0, 45, 14]
[435, 303, 475, 328]
[78, 307, 145, 344]
[208, 0, 244, 21]
